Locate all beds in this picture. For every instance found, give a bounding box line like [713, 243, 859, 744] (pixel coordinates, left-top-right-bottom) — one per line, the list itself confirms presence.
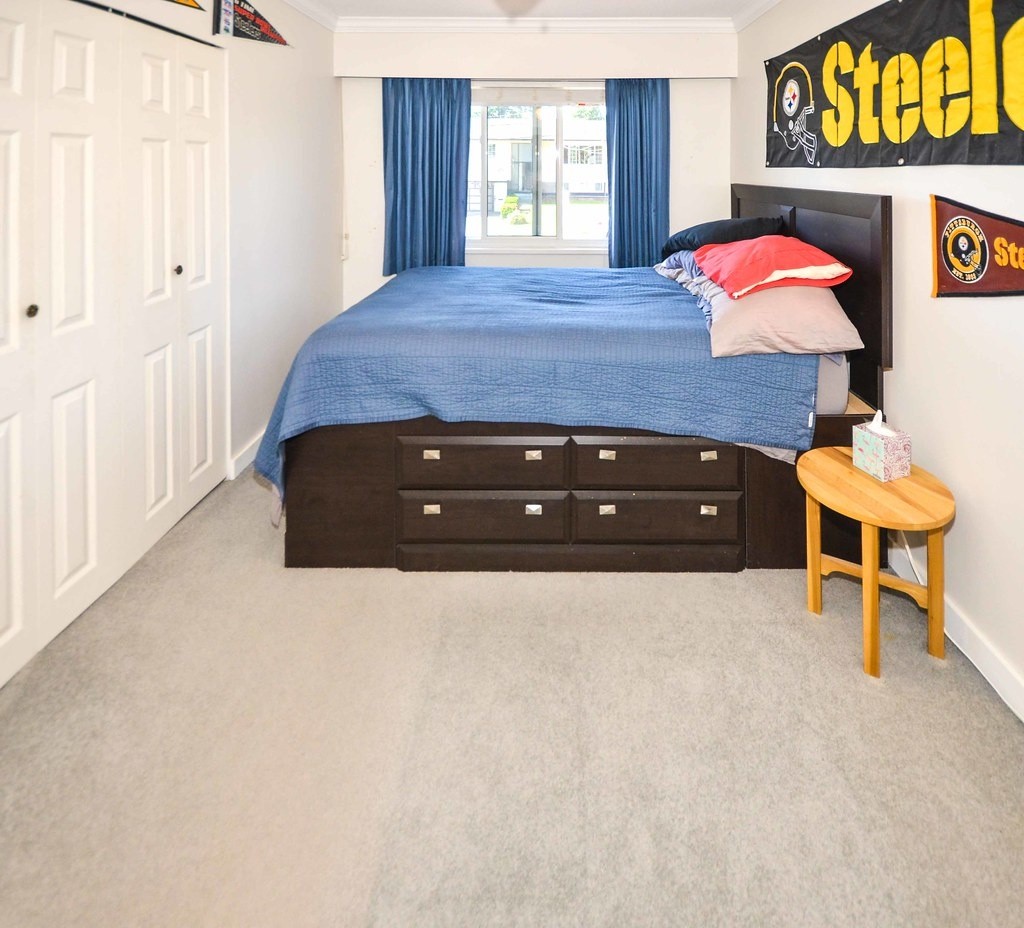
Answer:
[252, 174, 902, 574]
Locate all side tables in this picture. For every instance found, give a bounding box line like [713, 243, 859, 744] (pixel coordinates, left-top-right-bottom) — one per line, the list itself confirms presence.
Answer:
[796, 444, 961, 680]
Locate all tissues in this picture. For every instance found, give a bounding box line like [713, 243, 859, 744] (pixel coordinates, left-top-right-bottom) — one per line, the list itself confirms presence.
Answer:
[851, 409, 911, 483]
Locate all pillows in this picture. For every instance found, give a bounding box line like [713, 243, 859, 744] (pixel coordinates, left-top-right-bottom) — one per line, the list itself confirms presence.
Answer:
[692, 275, 865, 360]
[652, 262, 703, 296]
[656, 211, 785, 261]
[691, 233, 857, 301]
[661, 250, 705, 279]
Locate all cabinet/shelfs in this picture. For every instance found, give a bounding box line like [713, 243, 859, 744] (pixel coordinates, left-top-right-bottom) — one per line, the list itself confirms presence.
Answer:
[0, 0, 227, 693]
